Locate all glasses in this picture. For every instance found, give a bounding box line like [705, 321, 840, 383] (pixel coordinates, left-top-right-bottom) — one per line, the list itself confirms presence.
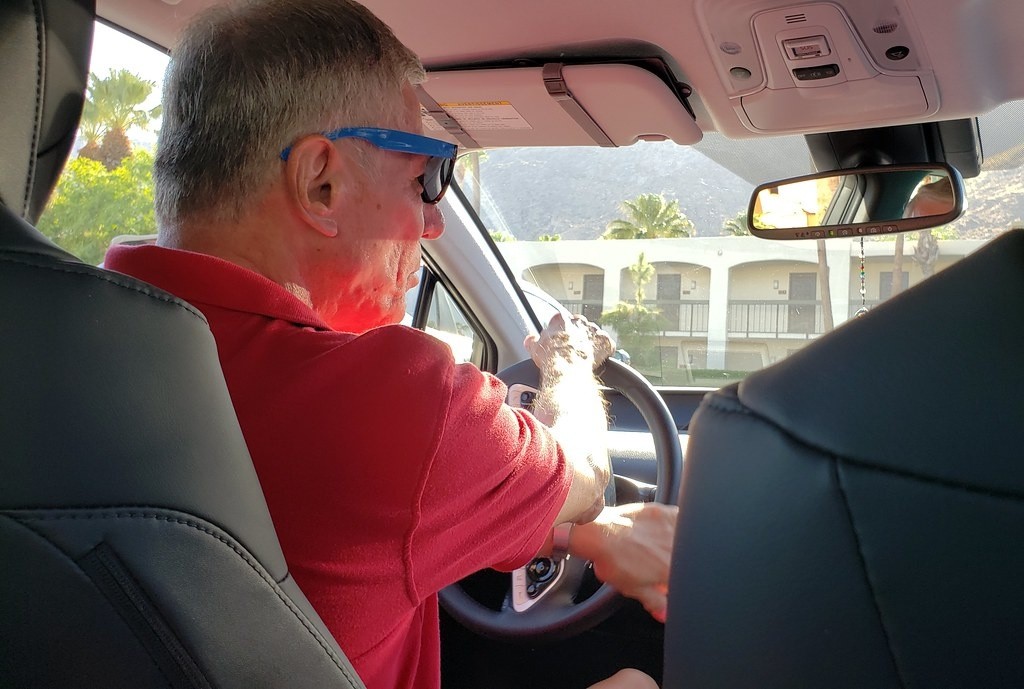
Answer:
[280, 127, 458, 205]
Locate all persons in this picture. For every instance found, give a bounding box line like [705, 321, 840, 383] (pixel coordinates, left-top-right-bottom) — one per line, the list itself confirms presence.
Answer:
[95, 1, 679, 689]
[903, 177, 953, 219]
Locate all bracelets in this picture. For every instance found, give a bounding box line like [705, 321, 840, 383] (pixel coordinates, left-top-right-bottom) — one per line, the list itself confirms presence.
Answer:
[552, 522, 573, 560]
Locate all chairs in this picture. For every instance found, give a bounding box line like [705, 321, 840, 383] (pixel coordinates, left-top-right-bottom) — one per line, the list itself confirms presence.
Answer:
[0, 0, 367, 689]
[662, 228, 1024, 689]
